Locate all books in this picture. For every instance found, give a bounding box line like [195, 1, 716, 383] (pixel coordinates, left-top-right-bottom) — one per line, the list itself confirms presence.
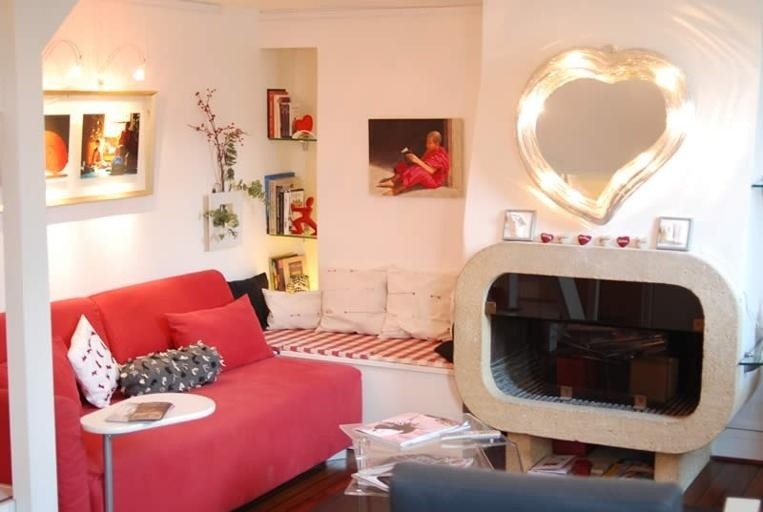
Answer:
[339, 413, 510, 496]
[264, 173, 307, 235]
[589, 446, 653, 479]
[267, 91, 301, 137]
[531, 456, 576, 477]
[269, 254, 307, 293]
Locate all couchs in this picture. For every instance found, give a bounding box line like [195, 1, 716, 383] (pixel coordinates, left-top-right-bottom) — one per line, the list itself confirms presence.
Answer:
[0, 270, 363, 512]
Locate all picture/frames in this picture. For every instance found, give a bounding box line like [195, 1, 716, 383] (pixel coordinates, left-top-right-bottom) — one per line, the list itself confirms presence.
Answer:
[656, 217, 692, 251]
[503, 210, 537, 241]
[43, 90, 158, 207]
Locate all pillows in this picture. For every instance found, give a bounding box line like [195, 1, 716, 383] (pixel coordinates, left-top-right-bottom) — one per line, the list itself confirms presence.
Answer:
[226, 272, 270, 331]
[67, 313, 121, 409]
[165, 293, 275, 372]
[377, 263, 458, 342]
[118, 340, 227, 396]
[261, 288, 324, 330]
[315, 266, 387, 335]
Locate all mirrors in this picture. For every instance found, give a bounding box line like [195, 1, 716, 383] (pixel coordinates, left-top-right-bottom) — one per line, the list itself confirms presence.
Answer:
[514, 45, 694, 225]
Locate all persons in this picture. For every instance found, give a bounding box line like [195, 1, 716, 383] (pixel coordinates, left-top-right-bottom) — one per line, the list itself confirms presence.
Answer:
[376, 132, 448, 196]
